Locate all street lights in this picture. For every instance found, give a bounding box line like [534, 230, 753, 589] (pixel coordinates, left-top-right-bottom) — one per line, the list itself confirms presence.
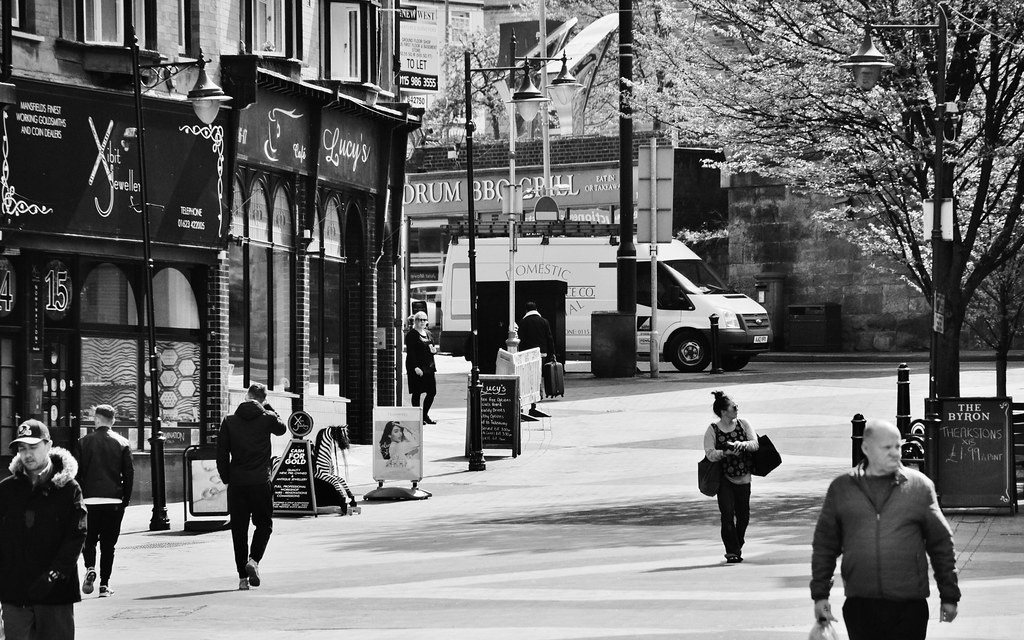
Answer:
[123, 20, 237, 539]
[503, 29, 584, 356]
[837, 0, 950, 516]
[461, 49, 550, 475]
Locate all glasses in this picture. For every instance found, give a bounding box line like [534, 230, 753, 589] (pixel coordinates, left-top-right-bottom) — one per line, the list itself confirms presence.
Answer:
[417, 318, 427, 321]
[725, 405, 738, 411]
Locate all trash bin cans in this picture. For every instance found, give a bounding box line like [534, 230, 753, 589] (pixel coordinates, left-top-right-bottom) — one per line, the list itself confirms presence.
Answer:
[591, 311, 637, 378]
[786, 303, 842, 352]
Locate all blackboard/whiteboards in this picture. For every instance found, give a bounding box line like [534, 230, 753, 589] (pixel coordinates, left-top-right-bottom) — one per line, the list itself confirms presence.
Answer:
[465, 374, 521, 449]
[924, 396, 1015, 508]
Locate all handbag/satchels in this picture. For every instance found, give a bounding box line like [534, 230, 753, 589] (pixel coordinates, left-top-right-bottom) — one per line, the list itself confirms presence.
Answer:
[807, 610, 839, 640]
[697, 424, 723, 497]
[738, 420, 782, 477]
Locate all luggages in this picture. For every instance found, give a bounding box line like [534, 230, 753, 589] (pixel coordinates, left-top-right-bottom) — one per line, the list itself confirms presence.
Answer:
[543, 358, 564, 398]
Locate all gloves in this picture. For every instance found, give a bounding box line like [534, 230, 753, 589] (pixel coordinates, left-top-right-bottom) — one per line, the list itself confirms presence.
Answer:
[27, 570, 54, 602]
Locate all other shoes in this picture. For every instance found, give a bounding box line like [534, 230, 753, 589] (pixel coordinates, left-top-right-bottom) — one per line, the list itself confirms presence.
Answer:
[246, 557, 260, 586]
[239, 578, 249, 590]
[423, 415, 435, 425]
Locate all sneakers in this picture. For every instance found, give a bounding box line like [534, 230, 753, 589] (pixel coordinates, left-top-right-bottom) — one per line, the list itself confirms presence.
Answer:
[99, 585, 114, 597]
[82, 568, 96, 594]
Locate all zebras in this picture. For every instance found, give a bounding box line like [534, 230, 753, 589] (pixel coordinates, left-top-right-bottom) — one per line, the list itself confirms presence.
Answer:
[270, 423, 357, 516]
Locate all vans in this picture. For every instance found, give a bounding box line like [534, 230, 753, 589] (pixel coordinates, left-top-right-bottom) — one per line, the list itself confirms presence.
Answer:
[436, 220, 773, 376]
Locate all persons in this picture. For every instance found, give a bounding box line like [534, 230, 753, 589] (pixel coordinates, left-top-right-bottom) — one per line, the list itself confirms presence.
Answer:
[77, 405, 134, 597]
[405, 311, 438, 425]
[518, 302, 556, 362]
[704, 391, 759, 563]
[810, 419, 962, 640]
[216, 383, 286, 589]
[0, 419, 87, 640]
[379, 421, 419, 467]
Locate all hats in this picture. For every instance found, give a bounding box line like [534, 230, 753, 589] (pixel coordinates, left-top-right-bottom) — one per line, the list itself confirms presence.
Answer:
[8, 419, 50, 449]
